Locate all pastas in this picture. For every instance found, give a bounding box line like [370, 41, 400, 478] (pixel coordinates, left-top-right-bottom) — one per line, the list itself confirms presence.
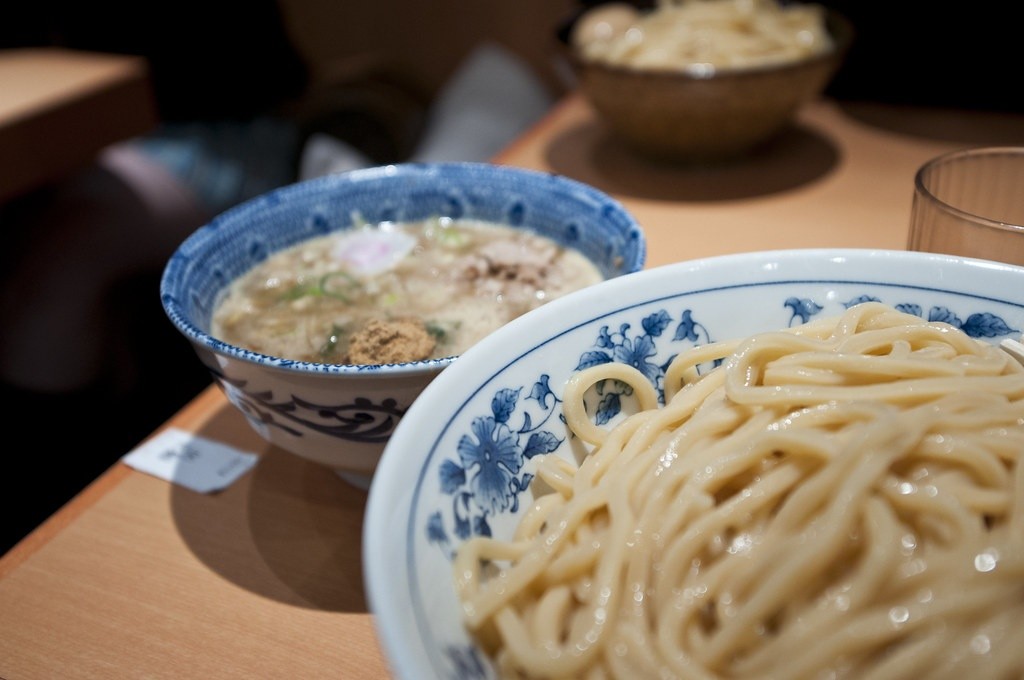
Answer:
[459, 301, 1024, 680]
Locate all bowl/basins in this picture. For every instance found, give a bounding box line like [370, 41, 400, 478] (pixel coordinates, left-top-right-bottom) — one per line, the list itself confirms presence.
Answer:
[361, 250, 1024, 680]
[163, 161, 647, 495]
[569, 47, 836, 159]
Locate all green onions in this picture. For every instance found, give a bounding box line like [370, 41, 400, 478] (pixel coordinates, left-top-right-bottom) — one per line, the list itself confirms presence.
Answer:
[277, 221, 461, 353]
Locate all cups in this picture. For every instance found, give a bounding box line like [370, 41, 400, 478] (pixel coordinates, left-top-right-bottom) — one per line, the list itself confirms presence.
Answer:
[907, 147, 1024, 265]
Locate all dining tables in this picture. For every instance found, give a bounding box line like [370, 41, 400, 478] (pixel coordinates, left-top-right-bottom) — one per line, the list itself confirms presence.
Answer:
[0, 87, 1023, 680]
